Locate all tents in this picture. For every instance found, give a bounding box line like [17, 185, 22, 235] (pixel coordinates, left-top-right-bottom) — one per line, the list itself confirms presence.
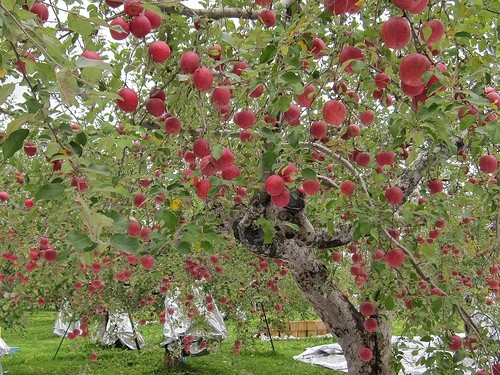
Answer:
[52, 269, 228, 356]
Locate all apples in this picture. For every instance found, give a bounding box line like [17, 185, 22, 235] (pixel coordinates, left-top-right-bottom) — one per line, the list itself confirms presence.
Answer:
[0, 0, 500, 375]
